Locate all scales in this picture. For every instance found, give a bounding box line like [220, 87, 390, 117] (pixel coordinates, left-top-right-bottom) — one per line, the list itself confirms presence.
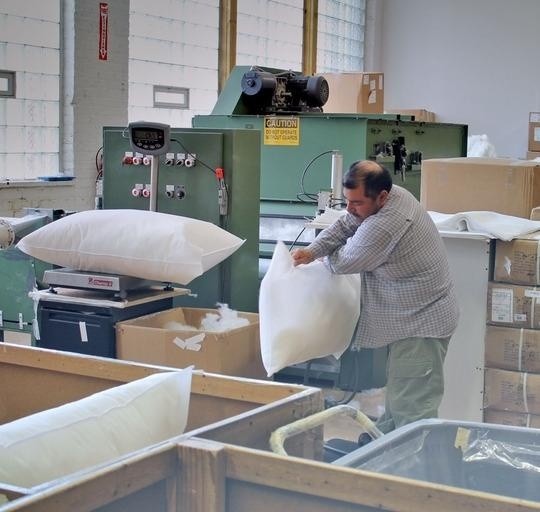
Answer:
[42, 121, 174, 302]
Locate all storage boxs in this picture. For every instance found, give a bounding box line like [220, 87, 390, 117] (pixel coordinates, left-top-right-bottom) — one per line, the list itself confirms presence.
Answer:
[418, 151, 537, 218]
[483, 281, 540, 328]
[121, 302, 259, 382]
[483, 366, 540, 410]
[489, 235, 540, 286]
[481, 410, 539, 430]
[482, 322, 539, 374]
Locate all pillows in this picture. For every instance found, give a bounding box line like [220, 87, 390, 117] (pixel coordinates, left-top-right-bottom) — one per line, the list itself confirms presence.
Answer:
[24, 211, 242, 279]
[259, 241, 360, 374]
[2, 368, 197, 482]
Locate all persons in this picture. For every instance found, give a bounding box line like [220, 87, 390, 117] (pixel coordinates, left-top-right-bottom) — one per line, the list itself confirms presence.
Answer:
[288, 159, 465, 448]
